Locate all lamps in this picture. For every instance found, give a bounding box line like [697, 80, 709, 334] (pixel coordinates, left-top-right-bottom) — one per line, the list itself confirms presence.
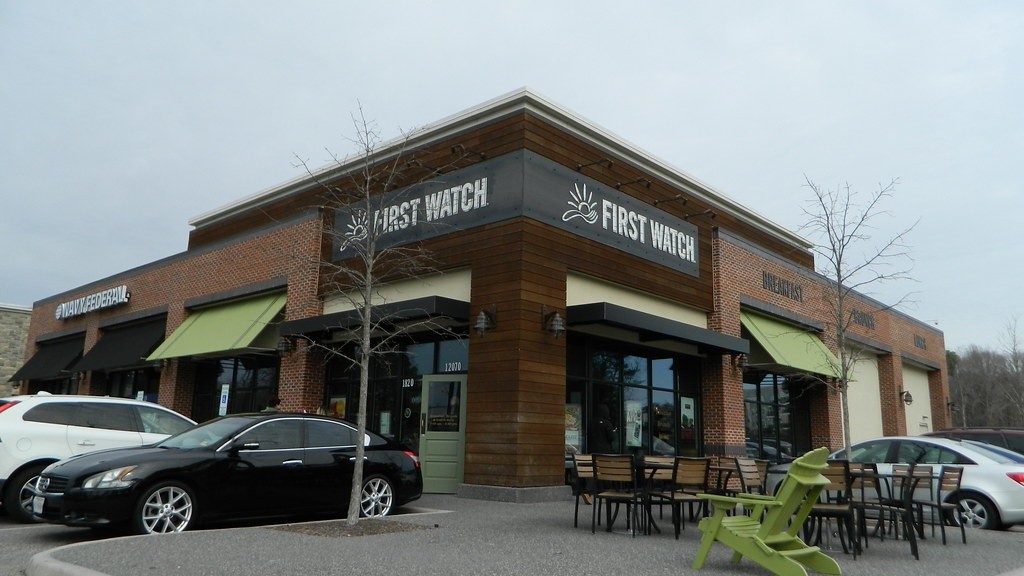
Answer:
[899, 385, 913, 407]
[277, 337, 297, 363]
[71, 370, 88, 385]
[731, 354, 751, 374]
[542, 304, 566, 340]
[472, 303, 497, 339]
[12, 380, 26, 392]
[946, 397, 961, 418]
[153, 358, 173, 376]
[832, 377, 849, 396]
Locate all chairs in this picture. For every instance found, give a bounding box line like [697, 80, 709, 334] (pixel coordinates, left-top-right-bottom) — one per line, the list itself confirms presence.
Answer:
[748, 458, 770, 517]
[913, 464, 967, 544]
[891, 466, 934, 538]
[718, 457, 742, 497]
[591, 453, 641, 538]
[571, 452, 603, 527]
[849, 462, 887, 536]
[636, 454, 683, 522]
[825, 462, 869, 551]
[734, 457, 767, 524]
[647, 455, 711, 540]
[804, 459, 857, 561]
[690, 446, 842, 576]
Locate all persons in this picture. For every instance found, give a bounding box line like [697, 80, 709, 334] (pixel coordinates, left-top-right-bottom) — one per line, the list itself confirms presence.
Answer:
[316, 406, 326, 415]
[260, 397, 282, 412]
[580, 403, 618, 506]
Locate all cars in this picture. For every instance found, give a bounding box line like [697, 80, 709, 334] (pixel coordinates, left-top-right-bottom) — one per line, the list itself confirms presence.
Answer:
[761, 434, 1024, 531]
[1, 394, 225, 527]
[31, 412, 424, 537]
[922, 425, 1024, 461]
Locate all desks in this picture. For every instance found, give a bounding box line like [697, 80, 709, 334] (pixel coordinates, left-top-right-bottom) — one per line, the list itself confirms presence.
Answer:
[849, 471, 940, 561]
[709, 466, 738, 517]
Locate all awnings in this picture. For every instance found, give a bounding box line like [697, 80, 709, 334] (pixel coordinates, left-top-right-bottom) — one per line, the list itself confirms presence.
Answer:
[7, 336, 84, 383]
[740, 311, 858, 382]
[145, 290, 288, 362]
[71, 319, 166, 374]
